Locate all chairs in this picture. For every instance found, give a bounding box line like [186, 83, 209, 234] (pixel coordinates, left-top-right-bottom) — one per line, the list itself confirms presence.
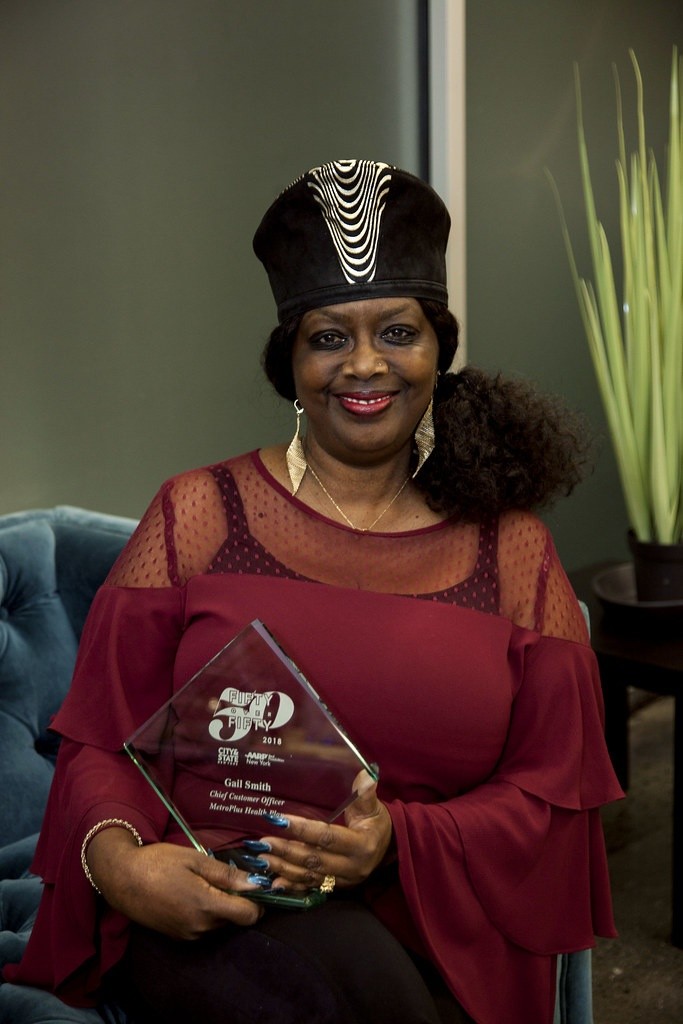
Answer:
[0, 507, 594, 1024]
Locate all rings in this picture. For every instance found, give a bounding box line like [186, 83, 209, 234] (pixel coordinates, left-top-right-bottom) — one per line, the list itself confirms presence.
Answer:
[320, 875, 335, 894]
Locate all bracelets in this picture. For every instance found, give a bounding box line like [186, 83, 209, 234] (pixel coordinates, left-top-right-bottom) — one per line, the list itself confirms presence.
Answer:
[81, 818, 142, 895]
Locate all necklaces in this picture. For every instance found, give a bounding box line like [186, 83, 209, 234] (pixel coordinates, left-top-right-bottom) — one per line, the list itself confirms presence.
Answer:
[301, 436, 418, 543]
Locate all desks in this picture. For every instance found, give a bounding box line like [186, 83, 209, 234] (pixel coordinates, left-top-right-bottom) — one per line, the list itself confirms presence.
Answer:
[589, 558, 683, 948]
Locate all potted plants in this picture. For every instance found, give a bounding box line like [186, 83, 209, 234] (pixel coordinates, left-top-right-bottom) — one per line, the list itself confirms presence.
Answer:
[543, 44, 683, 574]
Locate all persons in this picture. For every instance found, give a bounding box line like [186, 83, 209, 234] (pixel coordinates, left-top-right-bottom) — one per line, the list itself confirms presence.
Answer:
[0, 159, 628, 1024]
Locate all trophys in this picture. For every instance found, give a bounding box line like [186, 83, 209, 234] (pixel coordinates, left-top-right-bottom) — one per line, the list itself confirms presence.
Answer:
[124, 616, 379, 907]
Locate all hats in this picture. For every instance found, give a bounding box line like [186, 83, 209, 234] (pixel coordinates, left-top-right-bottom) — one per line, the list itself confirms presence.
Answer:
[253, 159, 451, 319]
